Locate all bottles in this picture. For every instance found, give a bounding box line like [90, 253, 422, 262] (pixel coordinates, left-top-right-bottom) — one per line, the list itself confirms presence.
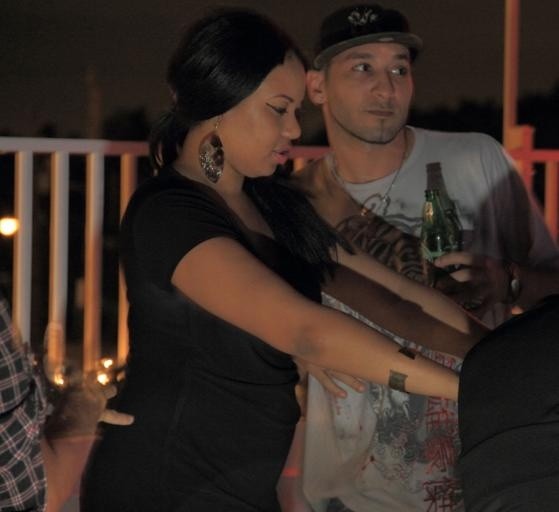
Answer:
[421, 185, 463, 283]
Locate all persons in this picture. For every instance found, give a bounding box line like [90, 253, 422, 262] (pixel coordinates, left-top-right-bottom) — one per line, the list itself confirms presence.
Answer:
[451, 291, 559, 512]
[284, 0, 559, 512]
[75, 8, 492, 512]
[1, 291, 47, 512]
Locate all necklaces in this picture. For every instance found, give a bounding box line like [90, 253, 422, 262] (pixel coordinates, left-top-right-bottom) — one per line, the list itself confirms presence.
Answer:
[316, 123, 408, 222]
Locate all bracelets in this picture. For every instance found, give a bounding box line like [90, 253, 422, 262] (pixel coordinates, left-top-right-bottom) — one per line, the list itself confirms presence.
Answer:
[388, 343, 418, 392]
[499, 260, 522, 301]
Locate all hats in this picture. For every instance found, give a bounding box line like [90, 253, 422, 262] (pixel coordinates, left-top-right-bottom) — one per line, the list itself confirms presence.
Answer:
[311, 3, 423, 69]
